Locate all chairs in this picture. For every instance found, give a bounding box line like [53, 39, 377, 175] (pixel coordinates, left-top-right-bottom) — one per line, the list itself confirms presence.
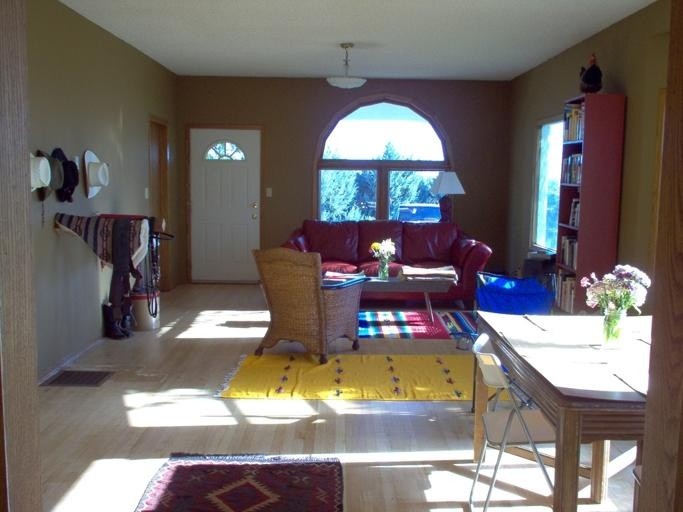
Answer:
[471, 270, 557, 415]
[469, 332, 594, 512]
[250, 247, 371, 364]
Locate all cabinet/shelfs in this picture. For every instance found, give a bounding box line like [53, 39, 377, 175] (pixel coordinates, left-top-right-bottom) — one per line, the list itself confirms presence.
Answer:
[553, 92, 626, 316]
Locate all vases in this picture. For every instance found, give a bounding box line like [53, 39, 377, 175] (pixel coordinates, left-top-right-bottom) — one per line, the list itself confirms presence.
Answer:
[377, 258, 388, 280]
[601, 309, 628, 350]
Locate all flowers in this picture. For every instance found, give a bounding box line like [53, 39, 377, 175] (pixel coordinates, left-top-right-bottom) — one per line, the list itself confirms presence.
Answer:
[580, 264, 651, 343]
[367, 237, 396, 280]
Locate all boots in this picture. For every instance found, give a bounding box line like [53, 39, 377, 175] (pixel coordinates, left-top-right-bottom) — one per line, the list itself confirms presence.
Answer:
[104, 303, 125, 341]
[122, 312, 134, 338]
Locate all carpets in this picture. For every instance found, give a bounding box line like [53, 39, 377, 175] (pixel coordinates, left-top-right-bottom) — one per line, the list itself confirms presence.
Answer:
[133, 452, 344, 512]
[355, 301, 478, 340]
[212, 352, 517, 402]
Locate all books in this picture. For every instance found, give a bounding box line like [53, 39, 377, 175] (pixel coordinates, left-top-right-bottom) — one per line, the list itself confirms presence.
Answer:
[562, 154, 581, 185]
[560, 236, 578, 271]
[569, 199, 580, 228]
[556, 269, 574, 314]
[323, 270, 366, 285]
[563, 103, 584, 141]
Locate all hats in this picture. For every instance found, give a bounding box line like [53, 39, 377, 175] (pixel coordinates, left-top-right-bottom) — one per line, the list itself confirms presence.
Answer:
[84, 149, 109, 200]
[51, 147, 80, 203]
[28, 152, 51, 192]
[36, 148, 65, 202]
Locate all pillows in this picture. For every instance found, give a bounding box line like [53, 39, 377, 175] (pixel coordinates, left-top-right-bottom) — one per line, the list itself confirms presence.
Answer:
[303, 220, 359, 265]
[358, 224, 403, 263]
[403, 222, 460, 263]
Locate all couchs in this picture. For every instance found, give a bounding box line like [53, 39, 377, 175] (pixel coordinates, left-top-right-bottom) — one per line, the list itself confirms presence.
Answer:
[279, 219, 492, 311]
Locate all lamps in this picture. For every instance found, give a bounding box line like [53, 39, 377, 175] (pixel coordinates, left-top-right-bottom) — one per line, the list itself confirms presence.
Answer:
[325, 42, 367, 89]
[430, 171, 466, 223]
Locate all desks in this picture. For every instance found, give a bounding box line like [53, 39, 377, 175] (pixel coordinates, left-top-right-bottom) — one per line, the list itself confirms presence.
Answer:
[474, 308, 653, 512]
